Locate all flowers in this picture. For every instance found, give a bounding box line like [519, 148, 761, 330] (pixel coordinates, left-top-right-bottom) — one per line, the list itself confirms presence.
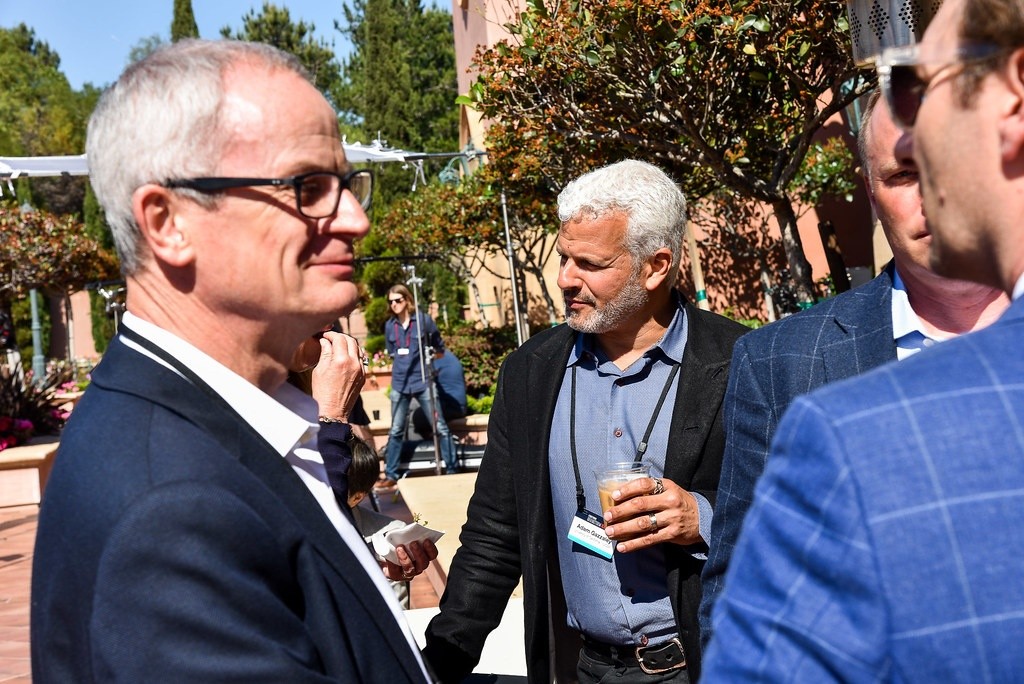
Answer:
[0, 359, 80, 452]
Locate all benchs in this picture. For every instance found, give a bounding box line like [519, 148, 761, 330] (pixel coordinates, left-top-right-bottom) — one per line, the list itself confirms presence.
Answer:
[451, 414, 490, 471]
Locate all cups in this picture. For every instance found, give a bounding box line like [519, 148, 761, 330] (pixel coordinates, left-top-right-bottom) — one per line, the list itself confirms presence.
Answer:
[591, 462, 656, 541]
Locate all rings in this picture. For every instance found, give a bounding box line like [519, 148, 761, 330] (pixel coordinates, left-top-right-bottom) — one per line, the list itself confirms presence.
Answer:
[402, 574, 415, 579]
[359, 355, 363, 359]
[653, 477, 664, 493]
[648, 513, 659, 532]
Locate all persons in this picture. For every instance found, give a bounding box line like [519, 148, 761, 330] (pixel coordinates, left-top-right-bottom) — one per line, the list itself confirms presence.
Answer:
[421, 160, 754, 684]
[30, 37, 467, 684]
[699, 0, 1024, 684]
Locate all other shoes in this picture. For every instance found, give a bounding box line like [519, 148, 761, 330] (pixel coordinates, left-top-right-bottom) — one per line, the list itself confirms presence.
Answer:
[377, 478, 397, 488]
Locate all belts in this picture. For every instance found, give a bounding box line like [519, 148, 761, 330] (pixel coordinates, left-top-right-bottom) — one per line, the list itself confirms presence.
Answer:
[579, 637, 687, 675]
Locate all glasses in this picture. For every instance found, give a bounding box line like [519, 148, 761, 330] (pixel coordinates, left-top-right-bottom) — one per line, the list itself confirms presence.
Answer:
[882, 45, 1024, 130]
[156, 170, 374, 219]
[388, 296, 405, 304]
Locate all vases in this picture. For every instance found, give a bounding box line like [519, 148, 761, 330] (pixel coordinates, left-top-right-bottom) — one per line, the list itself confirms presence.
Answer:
[0, 435, 59, 508]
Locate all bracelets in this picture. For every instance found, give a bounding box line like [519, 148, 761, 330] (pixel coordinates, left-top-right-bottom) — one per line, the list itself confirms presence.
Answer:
[318, 415, 354, 441]
